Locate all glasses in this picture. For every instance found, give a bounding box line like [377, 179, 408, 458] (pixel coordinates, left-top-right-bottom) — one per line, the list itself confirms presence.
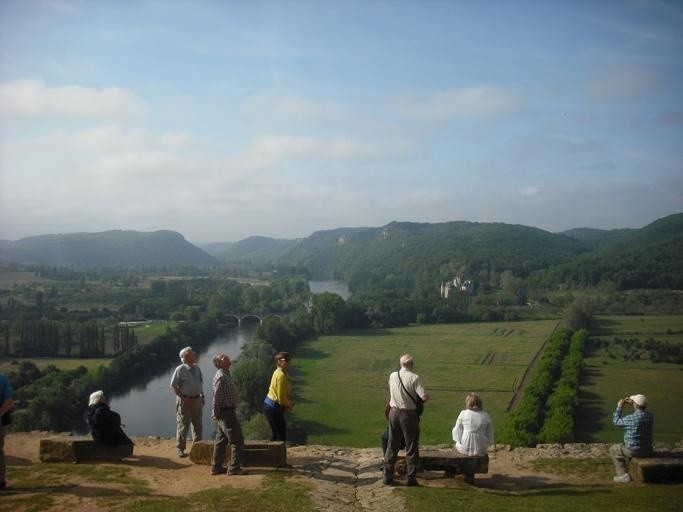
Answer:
[279, 358, 291, 362]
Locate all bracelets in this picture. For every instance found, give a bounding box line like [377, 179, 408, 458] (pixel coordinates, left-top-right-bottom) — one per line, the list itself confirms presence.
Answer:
[200, 395, 204, 398]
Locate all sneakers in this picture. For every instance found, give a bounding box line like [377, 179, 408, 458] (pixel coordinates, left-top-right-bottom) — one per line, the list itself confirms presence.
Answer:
[228, 468, 250, 475]
[177, 448, 185, 457]
[384, 479, 393, 485]
[406, 480, 417, 486]
[211, 468, 227, 475]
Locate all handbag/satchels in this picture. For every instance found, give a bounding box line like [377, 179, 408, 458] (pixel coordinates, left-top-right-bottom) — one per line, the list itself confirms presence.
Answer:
[416, 394, 424, 415]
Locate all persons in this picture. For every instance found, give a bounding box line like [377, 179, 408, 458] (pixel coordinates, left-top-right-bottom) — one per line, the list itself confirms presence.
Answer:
[86, 389, 133, 465]
[609, 393, 655, 483]
[382, 353, 429, 487]
[452, 392, 494, 458]
[382, 426, 405, 457]
[209, 352, 249, 476]
[0, 373, 14, 491]
[170, 346, 205, 458]
[264, 352, 295, 441]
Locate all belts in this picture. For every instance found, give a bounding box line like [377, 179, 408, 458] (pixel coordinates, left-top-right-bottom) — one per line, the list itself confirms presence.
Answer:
[183, 394, 200, 399]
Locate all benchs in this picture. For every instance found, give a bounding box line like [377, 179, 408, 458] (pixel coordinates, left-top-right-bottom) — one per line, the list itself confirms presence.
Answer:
[398, 450, 489, 483]
[190, 440, 286, 465]
[629, 456, 683, 483]
[39, 437, 132, 463]
